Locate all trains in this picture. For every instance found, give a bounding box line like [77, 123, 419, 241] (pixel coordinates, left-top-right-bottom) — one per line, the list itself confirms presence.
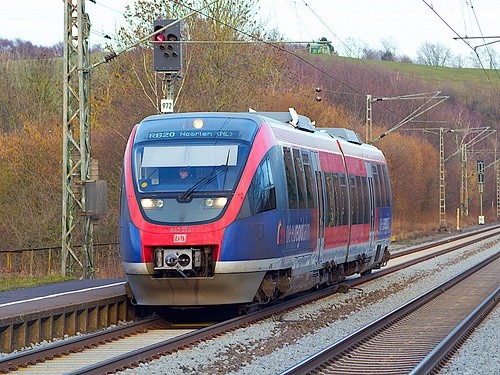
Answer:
[119, 112, 392, 316]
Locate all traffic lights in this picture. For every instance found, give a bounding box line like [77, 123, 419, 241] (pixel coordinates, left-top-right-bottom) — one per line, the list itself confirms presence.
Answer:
[154, 20, 180, 71]
